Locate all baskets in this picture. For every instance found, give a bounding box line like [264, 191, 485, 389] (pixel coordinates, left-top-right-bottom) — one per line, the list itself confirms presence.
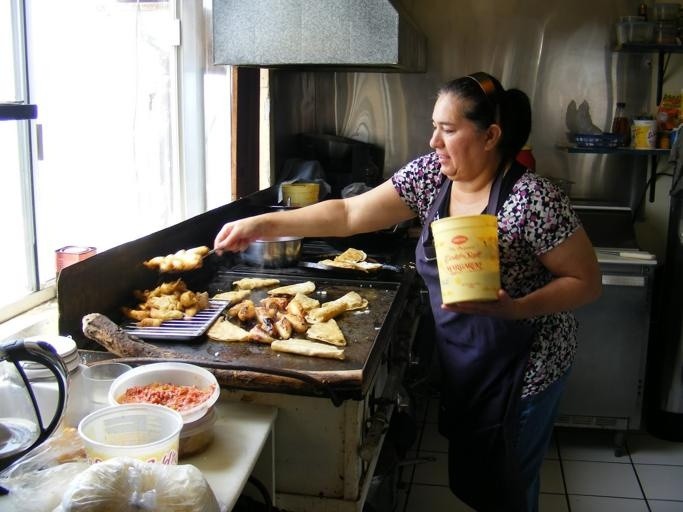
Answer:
[571, 132, 619, 147]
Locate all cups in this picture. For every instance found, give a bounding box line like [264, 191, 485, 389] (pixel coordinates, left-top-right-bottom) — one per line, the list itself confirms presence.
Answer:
[78, 404, 181, 469]
[630, 121, 657, 150]
[80, 363, 133, 411]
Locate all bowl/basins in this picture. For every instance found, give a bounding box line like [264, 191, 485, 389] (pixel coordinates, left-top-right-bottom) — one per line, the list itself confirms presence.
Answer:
[239, 236, 302, 269]
[576, 133, 616, 149]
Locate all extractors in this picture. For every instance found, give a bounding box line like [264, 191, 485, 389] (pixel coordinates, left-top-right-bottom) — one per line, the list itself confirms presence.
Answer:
[212, 1, 433, 75]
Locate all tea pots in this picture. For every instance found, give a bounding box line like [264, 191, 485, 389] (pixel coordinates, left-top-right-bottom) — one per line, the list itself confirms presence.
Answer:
[0, 336, 71, 471]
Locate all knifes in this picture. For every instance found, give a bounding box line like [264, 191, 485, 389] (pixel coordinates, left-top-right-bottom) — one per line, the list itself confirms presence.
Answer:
[597, 248, 657, 260]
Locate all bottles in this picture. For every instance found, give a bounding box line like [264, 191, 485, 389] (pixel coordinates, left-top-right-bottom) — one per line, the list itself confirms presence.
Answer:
[611, 102, 629, 144]
[638, 0, 649, 23]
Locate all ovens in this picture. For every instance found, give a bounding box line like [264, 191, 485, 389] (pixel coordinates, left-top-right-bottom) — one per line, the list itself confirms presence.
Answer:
[548, 263, 652, 429]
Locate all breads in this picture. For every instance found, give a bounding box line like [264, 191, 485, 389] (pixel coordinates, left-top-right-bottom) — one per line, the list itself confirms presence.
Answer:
[119, 245, 382, 361]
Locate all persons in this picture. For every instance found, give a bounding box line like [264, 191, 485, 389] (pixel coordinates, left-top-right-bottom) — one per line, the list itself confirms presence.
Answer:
[213, 72, 604, 512]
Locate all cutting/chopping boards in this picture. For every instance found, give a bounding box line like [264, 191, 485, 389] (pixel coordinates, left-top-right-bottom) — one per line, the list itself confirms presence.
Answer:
[591, 247, 657, 266]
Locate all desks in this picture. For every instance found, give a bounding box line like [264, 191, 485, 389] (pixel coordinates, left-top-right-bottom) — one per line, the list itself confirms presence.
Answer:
[176, 403, 279, 512]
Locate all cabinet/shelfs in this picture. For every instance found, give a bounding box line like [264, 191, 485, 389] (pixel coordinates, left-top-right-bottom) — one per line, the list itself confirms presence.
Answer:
[561, 41, 680, 214]
